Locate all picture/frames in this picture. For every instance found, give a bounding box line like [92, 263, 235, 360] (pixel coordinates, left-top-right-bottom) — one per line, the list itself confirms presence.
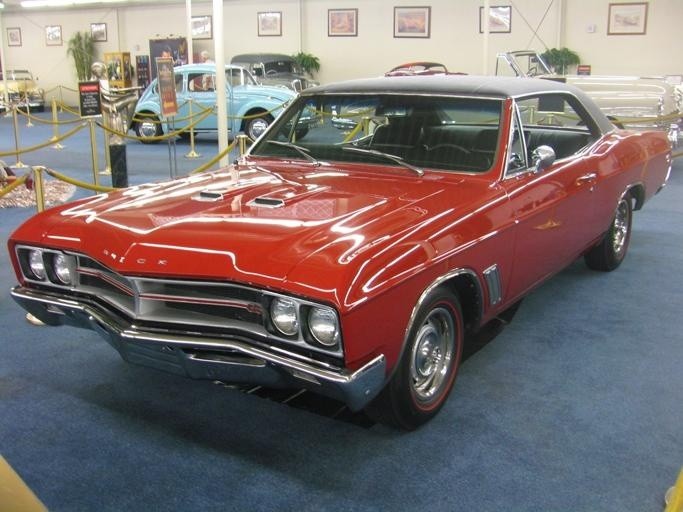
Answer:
[90, 22, 107, 42]
[257, 11, 282, 36]
[328, 8, 358, 37]
[46, 24, 63, 44]
[479, 5, 512, 33]
[6, 28, 22, 45]
[393, 6, 431, 38]
[606, 2, 648, 34]
[191, 15, 213, 40]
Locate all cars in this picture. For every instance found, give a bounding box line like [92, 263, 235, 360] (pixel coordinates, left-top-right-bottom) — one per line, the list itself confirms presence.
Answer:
[385, 60, 471, 76]
[227, 52, 321, 93]
[129, 63, 322, 145]
[0, 67, 46, 115]
[5, 75, 673, 423]
[330, 50, 683, 160]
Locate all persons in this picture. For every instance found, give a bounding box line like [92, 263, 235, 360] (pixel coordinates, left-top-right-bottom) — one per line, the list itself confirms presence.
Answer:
[200, 51, 214, 91]
[161, 46, 172, 58]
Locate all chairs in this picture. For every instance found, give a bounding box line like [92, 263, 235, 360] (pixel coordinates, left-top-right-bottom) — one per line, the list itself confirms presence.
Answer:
[470, 127, 531, 171]
[366, 124, 424, 160]
